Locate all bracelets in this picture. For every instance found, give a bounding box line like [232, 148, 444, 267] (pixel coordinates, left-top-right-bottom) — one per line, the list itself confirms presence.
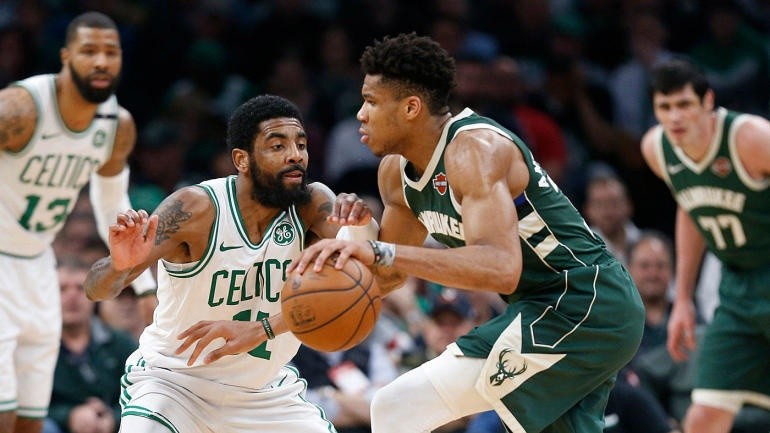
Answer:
[367, 239, 382, 265]
[376, 240, 396, 269]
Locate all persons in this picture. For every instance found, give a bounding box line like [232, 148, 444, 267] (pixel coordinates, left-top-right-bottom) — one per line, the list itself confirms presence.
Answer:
[638, 52, 770, 433]
[0, 0, 770, 433]
[0, 10, 158, 433]
[81, 93, 373, 433]
[174, 31, 647, 433]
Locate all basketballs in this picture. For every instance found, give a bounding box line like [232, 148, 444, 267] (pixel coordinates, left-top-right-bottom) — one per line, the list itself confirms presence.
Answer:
[280, 253, 382, 353]
